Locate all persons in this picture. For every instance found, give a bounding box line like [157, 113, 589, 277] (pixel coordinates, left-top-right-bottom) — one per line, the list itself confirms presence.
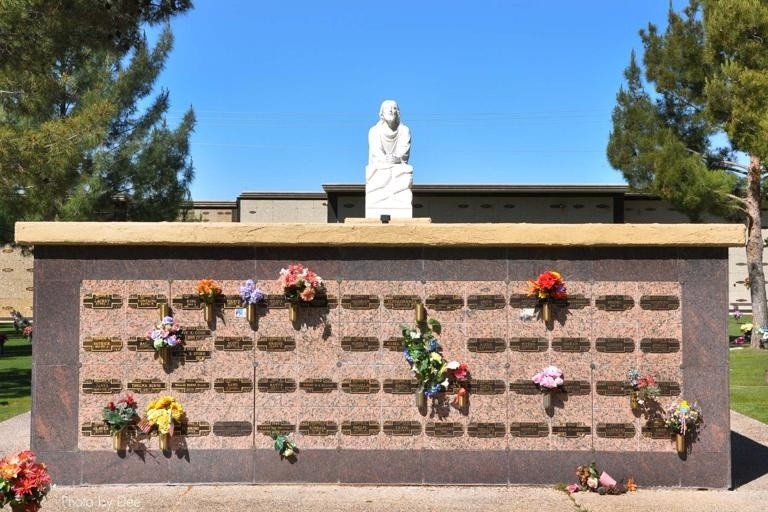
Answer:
[369, 100, 412, 170]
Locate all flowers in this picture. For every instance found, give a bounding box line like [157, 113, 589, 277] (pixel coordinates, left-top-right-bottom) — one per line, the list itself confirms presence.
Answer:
[1, 263, 703, 511]
